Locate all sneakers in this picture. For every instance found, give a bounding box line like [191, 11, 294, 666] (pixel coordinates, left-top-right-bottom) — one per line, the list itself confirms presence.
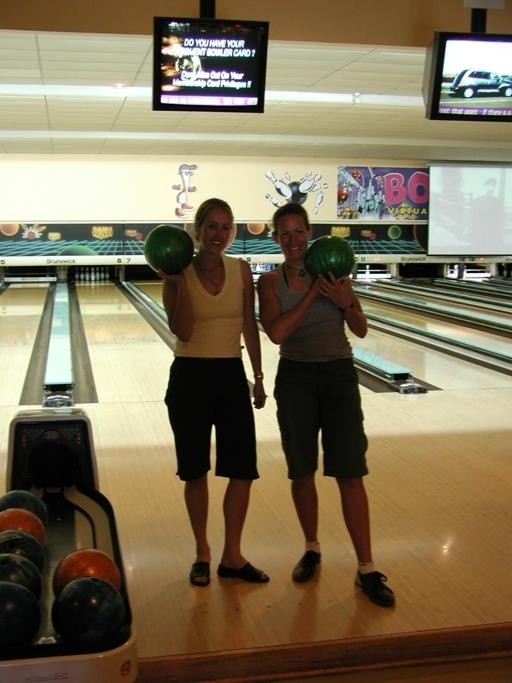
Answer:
[293, 551, 320, 583]
[355, 569, 395, 609]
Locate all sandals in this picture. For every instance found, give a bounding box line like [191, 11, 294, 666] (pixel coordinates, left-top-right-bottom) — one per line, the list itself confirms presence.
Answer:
[190, 562, 210, 587]
[217, 563, 269, 583]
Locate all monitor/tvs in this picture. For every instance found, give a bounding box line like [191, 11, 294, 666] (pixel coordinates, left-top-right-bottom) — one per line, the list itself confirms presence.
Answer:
[152, 15, 270, 113]
[422, 30, 512, 121]
